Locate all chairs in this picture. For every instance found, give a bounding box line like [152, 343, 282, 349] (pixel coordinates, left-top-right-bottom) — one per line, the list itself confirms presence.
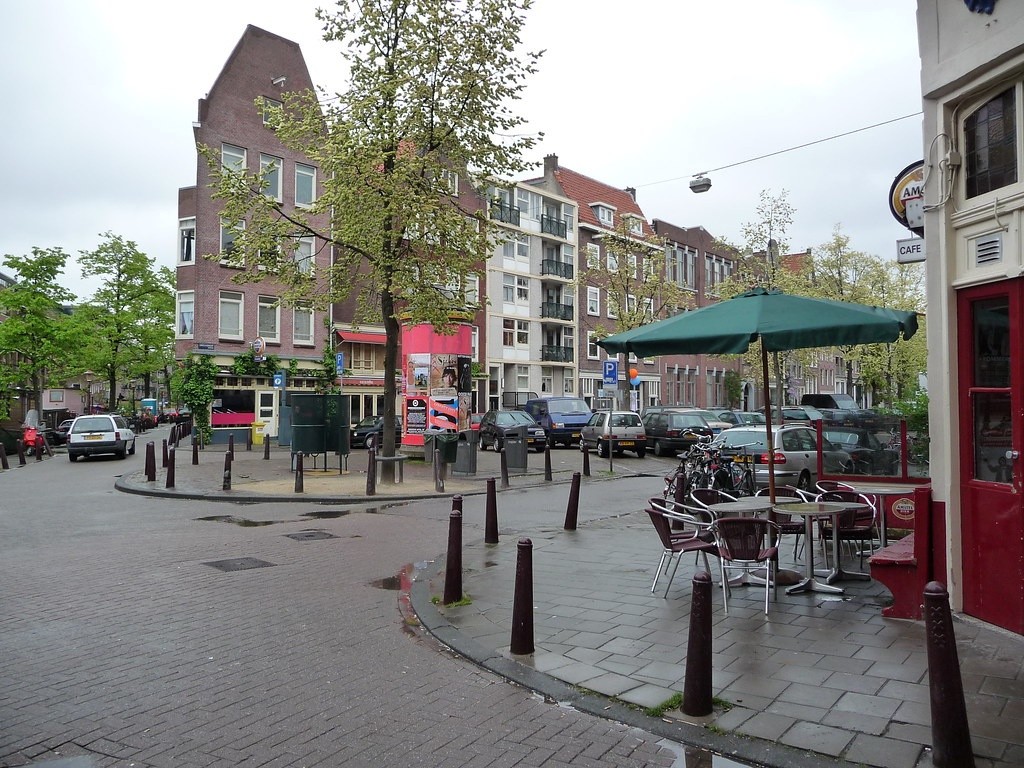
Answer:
[690, 488, 738, 524]
[756, 480, 880, 571]
[649, 497, 714, 571]
[710, 518, 783, 615]
[644, 508, 732, 598]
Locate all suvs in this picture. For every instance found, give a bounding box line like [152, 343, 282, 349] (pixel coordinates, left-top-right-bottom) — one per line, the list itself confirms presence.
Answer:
[348, 415, 402, 449]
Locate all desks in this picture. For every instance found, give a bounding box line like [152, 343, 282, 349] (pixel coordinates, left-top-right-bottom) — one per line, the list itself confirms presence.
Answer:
[707, 502, 776, 586]
[809, 501, 872, 584]
[735, 496, 802, 505]
[772, 502, 847, 596]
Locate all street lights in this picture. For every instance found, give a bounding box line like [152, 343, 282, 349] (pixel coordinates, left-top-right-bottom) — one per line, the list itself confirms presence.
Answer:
[129, 378, 138, 433]
[83, 368, 94, 414]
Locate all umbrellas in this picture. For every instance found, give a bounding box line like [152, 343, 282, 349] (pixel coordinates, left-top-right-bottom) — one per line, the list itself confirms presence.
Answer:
[596, 285, 918, 584]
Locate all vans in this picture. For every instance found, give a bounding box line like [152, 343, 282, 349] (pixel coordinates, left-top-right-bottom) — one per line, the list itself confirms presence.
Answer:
[801, 393, 862, 411]
[525, 396, 598, 449]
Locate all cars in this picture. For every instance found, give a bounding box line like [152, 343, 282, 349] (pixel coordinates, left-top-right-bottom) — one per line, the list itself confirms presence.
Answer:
[141, 409, 193, 428]
[578, 410, 647, 459]
[750, 404, 825, 424]
[809, 426, 900, 476]
[844, 401, 925, 426]
[711, 425, 856, 492]
[478, 408, 547, 453]
[640, 411, 714, 458]
[718, 411, 774, 428]
[471, 413, 485, 429]
[52, 419, 74, 444]
[66, 411, 136, 461]
[683, 410, 732, 437]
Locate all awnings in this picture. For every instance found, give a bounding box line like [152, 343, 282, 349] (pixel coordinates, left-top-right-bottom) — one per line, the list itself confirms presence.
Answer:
[334, 379, 384, 386]
[336, 331, 400, 345]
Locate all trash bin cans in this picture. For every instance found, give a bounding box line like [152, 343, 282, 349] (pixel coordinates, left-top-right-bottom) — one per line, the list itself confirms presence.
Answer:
[504, 424, 528, 472]
[251, 422, 266, 444]
[424, 433, 434, 461]
[437, 434, 459, 463]
[451, 429, 479, 475]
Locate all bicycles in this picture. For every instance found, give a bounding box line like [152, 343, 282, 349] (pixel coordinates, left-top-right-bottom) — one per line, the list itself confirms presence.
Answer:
[663, 431, 764, 504]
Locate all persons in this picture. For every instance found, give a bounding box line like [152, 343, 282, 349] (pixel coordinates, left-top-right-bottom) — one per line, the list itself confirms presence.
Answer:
[416, 374, 427, 386]
[440, 366, 457, 390]
[145, 407, 153, 429]
[408, 362, 416, 396]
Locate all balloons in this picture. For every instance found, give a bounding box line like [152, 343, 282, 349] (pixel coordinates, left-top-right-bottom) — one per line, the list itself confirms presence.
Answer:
[630, 368, 638, 378]
[630, 376, 640, 386]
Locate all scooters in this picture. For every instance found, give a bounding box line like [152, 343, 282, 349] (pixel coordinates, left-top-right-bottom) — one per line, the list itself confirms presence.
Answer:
[17, 409, 46, 456]
